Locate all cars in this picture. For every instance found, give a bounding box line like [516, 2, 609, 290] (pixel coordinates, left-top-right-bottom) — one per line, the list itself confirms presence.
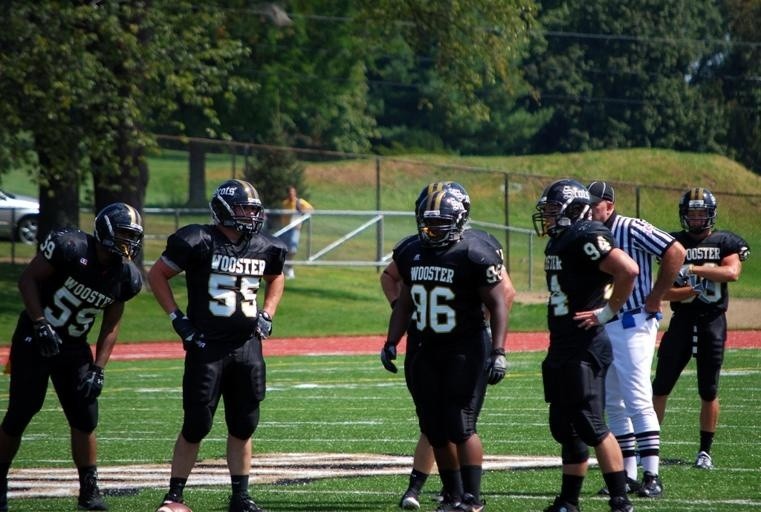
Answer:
[1, 190, 40, 246]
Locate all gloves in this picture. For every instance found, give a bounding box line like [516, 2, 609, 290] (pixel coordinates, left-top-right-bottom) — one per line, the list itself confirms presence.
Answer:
[483, 348, 508, 386]
[77, 365, 104, 404]
[380, 341, 399, 374]
[32, 319, 63, 357]
[168, 309, 206, 350]
[254, 311, 274, 339]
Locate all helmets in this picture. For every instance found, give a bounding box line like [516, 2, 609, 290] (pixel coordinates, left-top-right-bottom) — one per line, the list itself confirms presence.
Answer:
[417, 190, 464, 246]
[445, 180, 472, 223]
[531, 180, 590, 237]
[209, 179, 266, 238]
[678, 187, 718, 232]
[93, 202, 144, 261]
[415, 181, 451, 214]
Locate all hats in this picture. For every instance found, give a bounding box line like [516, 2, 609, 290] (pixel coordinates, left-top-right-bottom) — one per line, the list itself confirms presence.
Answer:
[586, 180, 615, 203]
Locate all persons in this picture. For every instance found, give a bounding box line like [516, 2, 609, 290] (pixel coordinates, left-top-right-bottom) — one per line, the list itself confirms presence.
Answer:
[652, 188, 751, 470]
[147, 179, 285, 512]
[276, 186, 314, 279]
[380, 180, 516, 511]
[587, 180, 686, 497]
[532, 179, 639, 512]
[0, 203, 142, 512]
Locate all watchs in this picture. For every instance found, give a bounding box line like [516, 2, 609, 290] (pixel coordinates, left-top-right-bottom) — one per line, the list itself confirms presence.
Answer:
[259, 310, 272, 323]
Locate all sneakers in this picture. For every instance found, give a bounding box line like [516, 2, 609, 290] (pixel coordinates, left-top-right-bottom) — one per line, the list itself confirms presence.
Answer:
[434, 496, 462, 512]
[694, 449, 713, 470]
[399, 487, 421, 510]
[543, 495, 581, 512]
[637, 471, 664, 497]
[448, 492, 487, 512]
[599, 476, 640, 496]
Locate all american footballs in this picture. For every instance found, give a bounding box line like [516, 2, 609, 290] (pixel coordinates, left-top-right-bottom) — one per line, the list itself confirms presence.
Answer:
[156, 502, 194, 512]
